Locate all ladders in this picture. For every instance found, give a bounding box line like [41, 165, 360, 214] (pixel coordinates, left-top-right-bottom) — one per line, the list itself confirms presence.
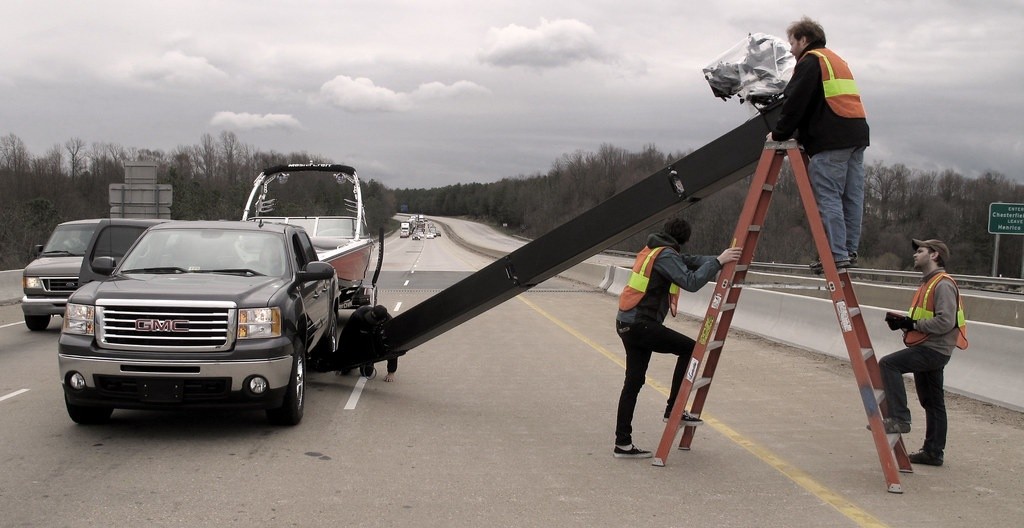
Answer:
[654, 132, 914, 496]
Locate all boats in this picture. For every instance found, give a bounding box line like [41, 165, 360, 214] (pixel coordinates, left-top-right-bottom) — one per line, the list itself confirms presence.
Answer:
[236, 162, 375, 291]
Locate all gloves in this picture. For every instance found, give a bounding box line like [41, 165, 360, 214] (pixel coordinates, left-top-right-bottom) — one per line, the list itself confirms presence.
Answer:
[885, 310, 914, 330]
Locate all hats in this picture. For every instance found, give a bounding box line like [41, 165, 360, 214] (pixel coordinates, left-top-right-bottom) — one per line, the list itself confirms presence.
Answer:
[365, 305, 387, 324]
[912, 238, 950, 268]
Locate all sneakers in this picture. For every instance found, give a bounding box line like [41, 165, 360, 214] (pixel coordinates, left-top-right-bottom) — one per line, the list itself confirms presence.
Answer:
[909, 448, 943, 466]
[664, 406, 704, 426]
[866, 417, 911, 433]
[614, 444, 652, 458]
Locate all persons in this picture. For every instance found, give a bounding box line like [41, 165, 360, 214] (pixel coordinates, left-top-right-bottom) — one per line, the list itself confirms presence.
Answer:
[338, 304, 398, 383]
[765, 14, 873, 273]
[612, 216, 743, 459]
[865, 238, 969, 467]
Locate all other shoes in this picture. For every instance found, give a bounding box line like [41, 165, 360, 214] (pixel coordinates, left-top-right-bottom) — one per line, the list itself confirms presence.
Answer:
[849, 254, 857, 264]
[810, 260, 850, 272]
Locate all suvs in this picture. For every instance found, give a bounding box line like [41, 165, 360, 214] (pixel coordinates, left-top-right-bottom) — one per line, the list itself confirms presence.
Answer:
[21, 217, 117, 330]
[58, 220, 340, 425]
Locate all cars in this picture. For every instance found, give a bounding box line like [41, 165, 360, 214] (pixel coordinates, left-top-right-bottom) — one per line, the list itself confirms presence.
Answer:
[400, 215, 441, 240]
